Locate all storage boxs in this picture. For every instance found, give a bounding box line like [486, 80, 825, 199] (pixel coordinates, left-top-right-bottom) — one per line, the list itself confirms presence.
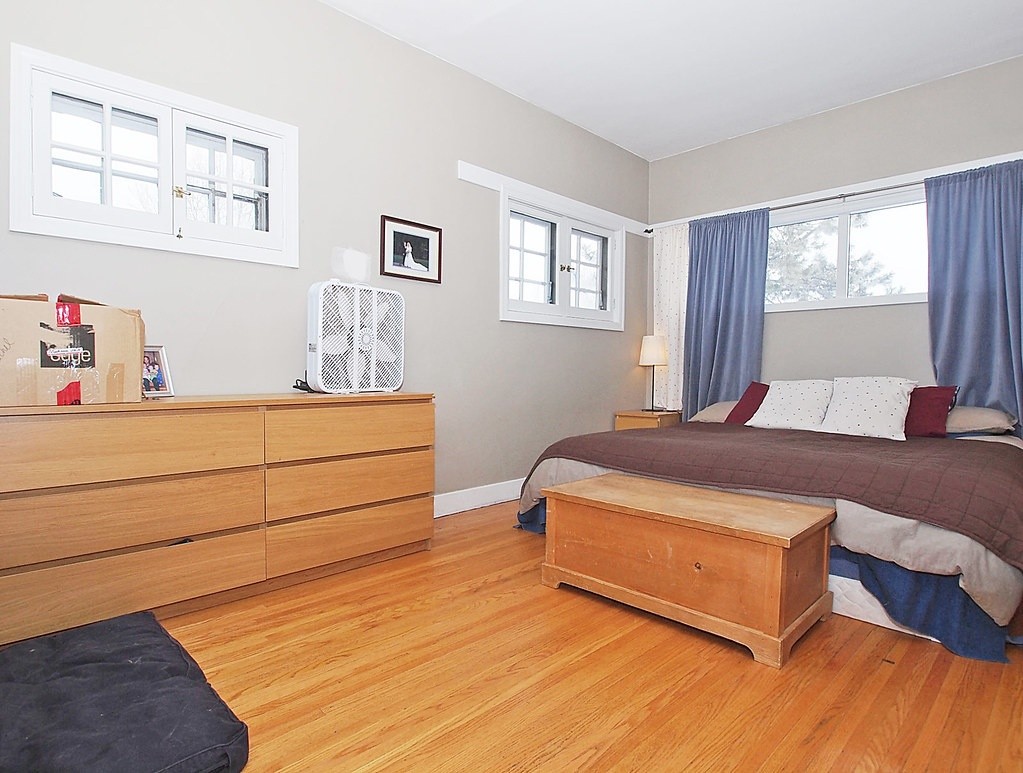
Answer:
[0, 293, 147, 407]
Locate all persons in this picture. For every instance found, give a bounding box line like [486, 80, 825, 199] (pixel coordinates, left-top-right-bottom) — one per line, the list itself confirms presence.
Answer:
[402, 241, 427, 270]
[143, 356, 163, 391]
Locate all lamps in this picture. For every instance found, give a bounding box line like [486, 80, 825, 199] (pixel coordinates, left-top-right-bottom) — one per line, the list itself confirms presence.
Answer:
[638, 334, 669, 412]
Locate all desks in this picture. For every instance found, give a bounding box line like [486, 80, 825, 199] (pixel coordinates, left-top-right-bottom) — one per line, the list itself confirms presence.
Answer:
[538, 472, 838, 670]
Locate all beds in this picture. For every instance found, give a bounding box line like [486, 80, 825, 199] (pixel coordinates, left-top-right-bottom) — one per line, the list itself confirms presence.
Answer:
[511, 373, 1023, 666]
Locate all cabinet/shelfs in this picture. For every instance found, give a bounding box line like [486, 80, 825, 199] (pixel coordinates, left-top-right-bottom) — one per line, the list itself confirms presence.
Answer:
[0, 391, 438, 651]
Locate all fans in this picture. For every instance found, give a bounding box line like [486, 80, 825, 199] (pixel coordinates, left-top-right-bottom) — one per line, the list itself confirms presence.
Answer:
[290, 280, 406, 396]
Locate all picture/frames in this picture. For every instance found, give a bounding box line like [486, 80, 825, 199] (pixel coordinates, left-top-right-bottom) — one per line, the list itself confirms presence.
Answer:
[141, 344, 176, 399]
[380, 215, 443, 284]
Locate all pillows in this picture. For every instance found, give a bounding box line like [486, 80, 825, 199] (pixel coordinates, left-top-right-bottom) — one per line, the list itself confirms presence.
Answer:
[687, 397, 741, 423]
[904, 385, 962, 437]
[724, 382, 769, 429]
[744, 378, 834, 432]
[816, 376, 920, 442]
[945, 404, 1020, 434]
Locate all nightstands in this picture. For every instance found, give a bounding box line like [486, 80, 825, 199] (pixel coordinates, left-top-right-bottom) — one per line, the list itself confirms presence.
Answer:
[614, 409, 683, 432]
